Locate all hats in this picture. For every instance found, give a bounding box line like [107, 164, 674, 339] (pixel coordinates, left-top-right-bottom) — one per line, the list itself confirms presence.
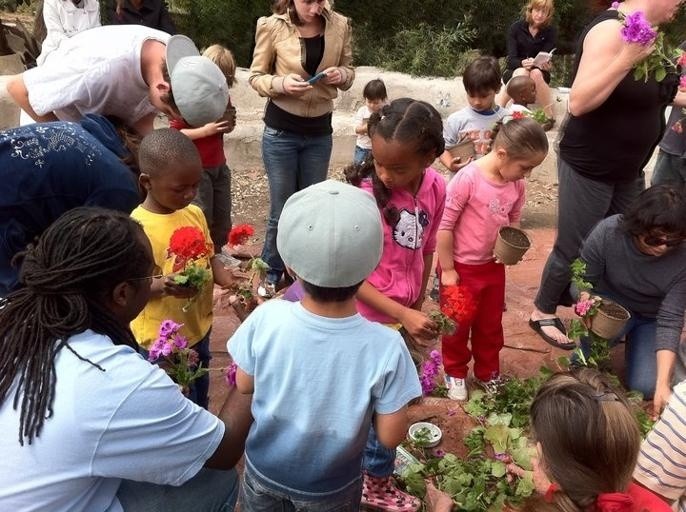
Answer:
[275, 180, 385, 289]
[163, 32, 230, 129]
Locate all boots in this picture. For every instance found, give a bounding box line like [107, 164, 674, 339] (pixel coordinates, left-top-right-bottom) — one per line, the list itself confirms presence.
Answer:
[360, 474, 422, 512]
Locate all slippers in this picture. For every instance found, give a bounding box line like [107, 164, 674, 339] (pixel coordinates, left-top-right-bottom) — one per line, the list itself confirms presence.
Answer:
[528, 308, 577, 351]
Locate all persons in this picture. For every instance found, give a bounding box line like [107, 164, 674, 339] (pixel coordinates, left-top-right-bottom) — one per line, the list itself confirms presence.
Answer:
[0, 0, 686, 512]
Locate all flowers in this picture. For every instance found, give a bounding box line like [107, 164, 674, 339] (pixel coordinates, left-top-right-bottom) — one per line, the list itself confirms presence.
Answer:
[417, 311, 452, 394]
[492, 108, 552, 134]
[164, 225, 212, 311]
[225, 221, 269, 296]
[604, 1, 686, 89]
[566, 256, 601, 341]
[443, 281, 476, 320]
[143, 317, 239, 397]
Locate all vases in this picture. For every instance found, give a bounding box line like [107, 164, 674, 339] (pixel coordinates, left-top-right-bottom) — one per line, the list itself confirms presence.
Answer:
[494, 225, 531, 267]
[448, 138, 478, 163]
[592, 297, 629, 340]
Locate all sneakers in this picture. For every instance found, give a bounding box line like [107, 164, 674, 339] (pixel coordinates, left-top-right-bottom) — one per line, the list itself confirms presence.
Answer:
[471, 370, 505, 393]
[443, 373, 467, 401]
[221, 241, 243, 257]
[257, 282, 275, 299]
[215, 247, 242, 268]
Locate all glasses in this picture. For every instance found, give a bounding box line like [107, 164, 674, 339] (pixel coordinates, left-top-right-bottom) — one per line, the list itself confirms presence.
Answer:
[127, 264, 163, 283]
